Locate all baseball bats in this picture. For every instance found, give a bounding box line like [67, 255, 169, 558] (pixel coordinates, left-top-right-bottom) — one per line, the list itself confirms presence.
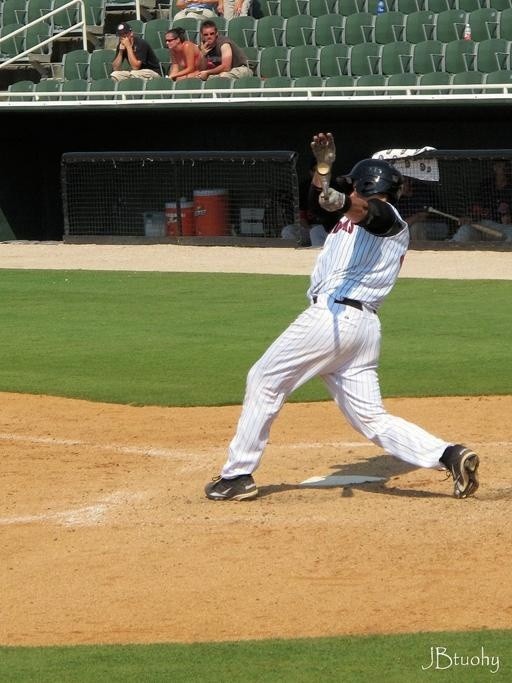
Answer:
[317, 163, 330, 201]
[427, 206, 504, 240]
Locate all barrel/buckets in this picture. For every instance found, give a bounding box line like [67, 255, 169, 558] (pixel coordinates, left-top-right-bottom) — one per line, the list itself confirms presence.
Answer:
[165, 201, 193, 236]
[193, 188, 231, 237]
[144, 209, 165, 236]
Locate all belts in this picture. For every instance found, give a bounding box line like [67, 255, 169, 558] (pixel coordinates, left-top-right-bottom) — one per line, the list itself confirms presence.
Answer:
[313, 295, 377, 315]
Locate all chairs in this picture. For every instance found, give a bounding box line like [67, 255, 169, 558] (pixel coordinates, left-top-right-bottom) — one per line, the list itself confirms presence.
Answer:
[1, 1, 511, 101]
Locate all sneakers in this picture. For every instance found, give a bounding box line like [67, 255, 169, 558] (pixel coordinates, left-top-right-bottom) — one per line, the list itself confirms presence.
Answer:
[440, 444, 480, 499]
[204, 474, 259, 501]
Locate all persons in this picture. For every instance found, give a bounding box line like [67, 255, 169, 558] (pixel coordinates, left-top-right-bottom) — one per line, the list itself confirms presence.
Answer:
[110, 23, 162, 80]
[472, 159, 512, 224]
[204, 132, 479, 502]
[193, 20, 254, 80]
[217, 0, 250, 20]
[395, 176, 428, 240]
[165, 27, 208, 82]
[173, 0, 218, 22]
[280, 157, 336, 247]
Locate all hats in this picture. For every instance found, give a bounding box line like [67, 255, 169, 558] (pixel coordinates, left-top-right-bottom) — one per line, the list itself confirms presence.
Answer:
[116, 22, 132, 35]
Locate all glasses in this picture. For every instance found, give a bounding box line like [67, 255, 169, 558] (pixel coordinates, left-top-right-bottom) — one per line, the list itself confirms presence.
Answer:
[166, 36, 179, 42]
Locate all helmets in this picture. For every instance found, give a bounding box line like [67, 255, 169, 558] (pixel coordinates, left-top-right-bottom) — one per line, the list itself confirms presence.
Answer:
[342, 158, 402, 201]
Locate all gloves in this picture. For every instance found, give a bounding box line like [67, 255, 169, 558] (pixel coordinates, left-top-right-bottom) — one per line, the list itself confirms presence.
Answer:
[319, 188, 352, 214]
